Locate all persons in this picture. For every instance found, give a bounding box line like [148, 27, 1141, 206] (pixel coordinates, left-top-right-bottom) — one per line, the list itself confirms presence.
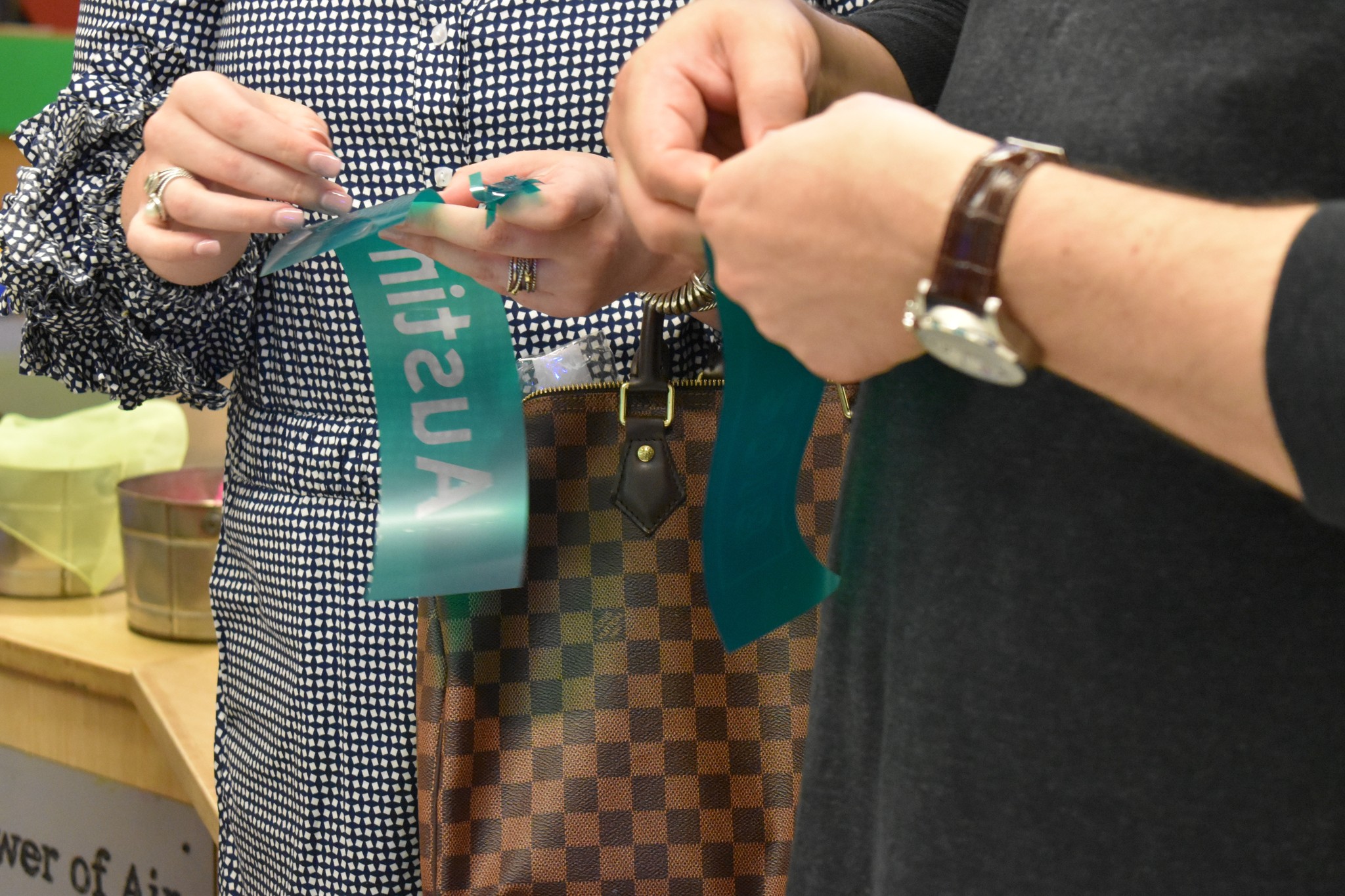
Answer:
[591, 0, 1344, 889]
[3, 0, 836, 896]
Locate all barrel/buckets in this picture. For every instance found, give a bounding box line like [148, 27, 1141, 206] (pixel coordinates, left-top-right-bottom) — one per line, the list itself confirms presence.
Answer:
[1, 443, 132, 600]
[117, 462, 243, 642]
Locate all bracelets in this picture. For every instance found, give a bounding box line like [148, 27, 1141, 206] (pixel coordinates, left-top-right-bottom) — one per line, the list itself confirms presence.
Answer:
[633, 270, 716, 321]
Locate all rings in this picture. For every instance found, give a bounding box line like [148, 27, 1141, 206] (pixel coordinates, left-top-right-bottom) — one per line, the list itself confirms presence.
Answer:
[500, 254, 546, 298]
[143, 168, 180, 225]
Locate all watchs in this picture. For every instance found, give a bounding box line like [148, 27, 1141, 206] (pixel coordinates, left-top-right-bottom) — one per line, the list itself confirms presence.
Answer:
[902, 131, 1069, 389]
